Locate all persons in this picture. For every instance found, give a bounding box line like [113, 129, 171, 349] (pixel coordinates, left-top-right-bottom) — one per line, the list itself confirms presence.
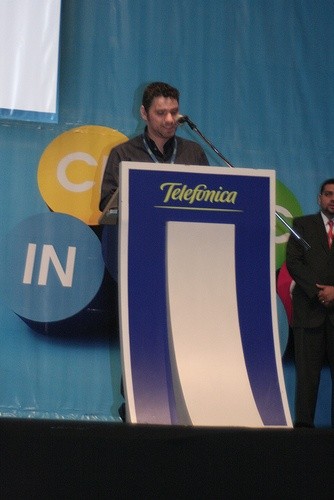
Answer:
[99, 82, 213, 422]
[285, 178, 334, 428]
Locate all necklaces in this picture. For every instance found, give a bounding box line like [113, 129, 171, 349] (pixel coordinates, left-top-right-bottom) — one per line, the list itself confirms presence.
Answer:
[139, 130, 176, 165]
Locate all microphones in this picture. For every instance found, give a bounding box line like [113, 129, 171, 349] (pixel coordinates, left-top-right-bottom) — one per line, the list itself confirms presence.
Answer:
[175, 115, 187, 125]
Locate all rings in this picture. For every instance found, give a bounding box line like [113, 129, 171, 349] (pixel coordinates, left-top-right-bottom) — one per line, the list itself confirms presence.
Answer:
[321, 299, 326, 303]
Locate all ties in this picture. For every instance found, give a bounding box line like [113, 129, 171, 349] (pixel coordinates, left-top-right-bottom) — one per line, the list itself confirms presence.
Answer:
[328, 221, 334, 250]
